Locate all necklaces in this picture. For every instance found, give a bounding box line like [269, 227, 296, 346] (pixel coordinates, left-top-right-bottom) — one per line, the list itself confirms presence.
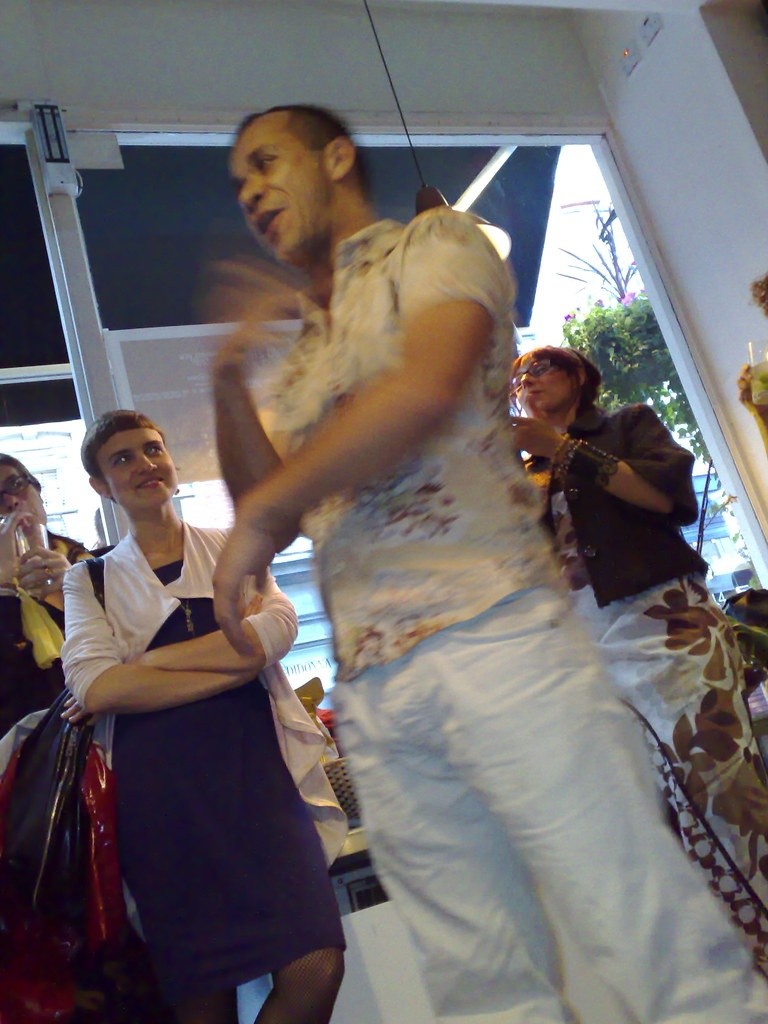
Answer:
[179, 599, 196, 632]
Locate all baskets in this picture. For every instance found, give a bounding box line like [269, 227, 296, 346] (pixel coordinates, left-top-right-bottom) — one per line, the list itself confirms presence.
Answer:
[321, 756, 359, 820]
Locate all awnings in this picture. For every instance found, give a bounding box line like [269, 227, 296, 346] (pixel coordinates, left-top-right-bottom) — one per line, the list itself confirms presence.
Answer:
[0, 146, 562, 426]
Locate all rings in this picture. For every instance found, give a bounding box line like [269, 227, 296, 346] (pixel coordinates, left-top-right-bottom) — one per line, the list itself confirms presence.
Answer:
[41, 559, 48, 568]
[43, 567, 53, 577]
[0, 516, 8, 525]
[46, 579, 52, 586]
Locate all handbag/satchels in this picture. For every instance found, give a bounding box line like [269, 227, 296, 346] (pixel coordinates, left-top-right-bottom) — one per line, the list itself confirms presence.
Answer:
[8, 557, 103, 926]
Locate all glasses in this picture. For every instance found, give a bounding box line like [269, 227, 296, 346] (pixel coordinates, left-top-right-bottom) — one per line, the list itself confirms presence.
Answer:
[0, 471, 32, 503]
[511, 357, 551, 387]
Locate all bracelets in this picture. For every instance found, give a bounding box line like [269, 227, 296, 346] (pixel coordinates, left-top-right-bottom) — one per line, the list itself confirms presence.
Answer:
[550, 432, 619, 492]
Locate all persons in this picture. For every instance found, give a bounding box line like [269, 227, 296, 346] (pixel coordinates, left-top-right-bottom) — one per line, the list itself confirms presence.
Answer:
[737, 272, 768, 452]
[211, 103, 768, 1024]
[0, 454, 97, 741]
[507, 347, 768, 881]
[57, 411, 349, 1024]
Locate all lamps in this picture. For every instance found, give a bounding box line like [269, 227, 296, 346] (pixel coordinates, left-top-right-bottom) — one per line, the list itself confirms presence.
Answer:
[363, 1, 513, 261]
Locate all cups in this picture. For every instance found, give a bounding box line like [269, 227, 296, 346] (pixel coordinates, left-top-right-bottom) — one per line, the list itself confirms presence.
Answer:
[748, 341, 768, 405]
[14, 524, 52, 588]
